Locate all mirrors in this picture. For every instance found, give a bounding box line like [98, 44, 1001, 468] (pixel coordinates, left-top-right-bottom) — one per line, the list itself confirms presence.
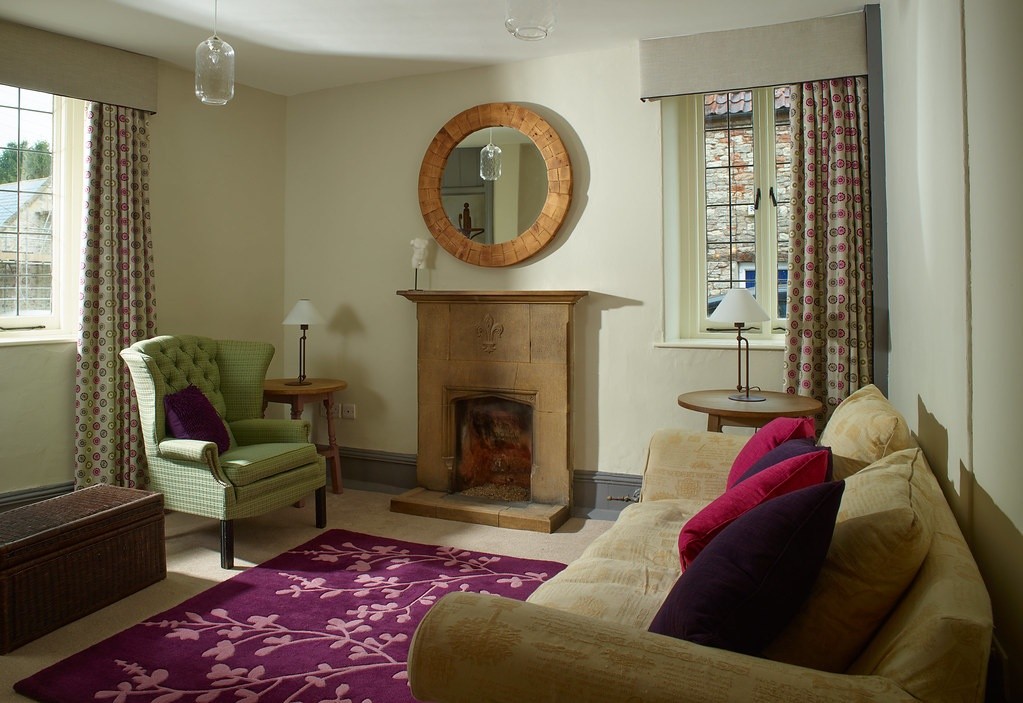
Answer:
[416, 100, 574, 267]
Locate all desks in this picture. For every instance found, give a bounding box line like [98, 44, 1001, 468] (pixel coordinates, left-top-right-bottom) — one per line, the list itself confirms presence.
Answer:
[678, 387, 823, 434]
[259, 377, 349, 495]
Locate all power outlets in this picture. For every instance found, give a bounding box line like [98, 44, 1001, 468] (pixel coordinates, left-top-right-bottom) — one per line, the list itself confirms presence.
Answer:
[320, 401, 356, 420]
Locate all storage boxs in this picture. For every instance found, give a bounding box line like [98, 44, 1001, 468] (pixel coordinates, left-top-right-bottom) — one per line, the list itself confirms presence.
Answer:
[1, 483, 170, 658]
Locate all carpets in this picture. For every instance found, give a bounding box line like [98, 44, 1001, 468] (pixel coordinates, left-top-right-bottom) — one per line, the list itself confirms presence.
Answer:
[12, 525, 569, 703]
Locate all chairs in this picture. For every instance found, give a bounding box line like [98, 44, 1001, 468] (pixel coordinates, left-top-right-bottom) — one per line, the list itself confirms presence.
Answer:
[118, 333, 331, 569]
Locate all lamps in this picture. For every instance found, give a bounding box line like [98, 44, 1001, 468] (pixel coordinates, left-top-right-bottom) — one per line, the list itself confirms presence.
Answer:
[708, 286, 771, 403]
[279, 297, 328, 386]
[193, 1, 235, 106]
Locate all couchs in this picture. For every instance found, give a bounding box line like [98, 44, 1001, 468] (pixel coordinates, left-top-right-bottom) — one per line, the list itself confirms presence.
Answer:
[401, 374, 998, 703]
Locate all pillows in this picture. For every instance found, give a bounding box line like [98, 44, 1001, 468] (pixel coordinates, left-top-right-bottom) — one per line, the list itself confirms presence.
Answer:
[774, 443, 930, 683]
[732, 436, 825, 493]
[723, 412, 810, 490]
[675, 447, 830, 581]
[644, 477, 850, 657]
[816, 380, 913, 480]
